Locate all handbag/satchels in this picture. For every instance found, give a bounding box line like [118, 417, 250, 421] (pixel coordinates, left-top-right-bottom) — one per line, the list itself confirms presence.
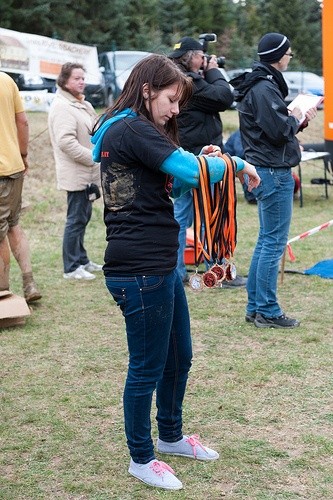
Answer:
[0, 290, 31, 328]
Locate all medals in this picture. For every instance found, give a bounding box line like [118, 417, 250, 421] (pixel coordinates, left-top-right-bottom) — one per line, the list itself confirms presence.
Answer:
[189, 261, 237, 291]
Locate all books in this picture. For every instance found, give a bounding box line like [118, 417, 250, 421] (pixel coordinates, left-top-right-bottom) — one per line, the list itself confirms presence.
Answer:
[287, 93, 324, 133]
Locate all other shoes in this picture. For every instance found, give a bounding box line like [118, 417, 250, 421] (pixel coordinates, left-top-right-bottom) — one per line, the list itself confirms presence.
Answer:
[245, 190, 256, 204]
[222, 275, 248, 288]
[63, 265, 95, 280]
[24, 283, 42, 302]
[83, 261, 103, 273]
[182, 274, 190, 283]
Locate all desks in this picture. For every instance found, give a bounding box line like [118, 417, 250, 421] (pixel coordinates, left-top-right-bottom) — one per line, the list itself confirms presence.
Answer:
[296, 149, 331, 207]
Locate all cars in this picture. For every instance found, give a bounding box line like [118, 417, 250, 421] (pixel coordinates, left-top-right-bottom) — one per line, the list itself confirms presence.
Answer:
[200, 69, 324, 102]
[14, 74, 104, 108]
[97, 52, 151, 108]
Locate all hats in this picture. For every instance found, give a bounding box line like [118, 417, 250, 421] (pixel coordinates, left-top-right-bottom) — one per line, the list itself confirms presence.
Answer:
[167, 37, 203, 58]
[257, 33, 290, 63]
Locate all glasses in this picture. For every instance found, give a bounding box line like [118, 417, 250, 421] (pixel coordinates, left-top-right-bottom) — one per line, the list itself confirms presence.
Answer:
[285, 52, 294, 56]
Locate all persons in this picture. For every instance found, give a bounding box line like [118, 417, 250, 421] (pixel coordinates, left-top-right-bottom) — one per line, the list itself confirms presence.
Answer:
[222, 126, 298, 204]
[0, 70, 42, 303]
[83, 56, 263, 489]
[47, 63, 103, 281]
[228, 33, 317, 329]
[163, 36, 249, 286]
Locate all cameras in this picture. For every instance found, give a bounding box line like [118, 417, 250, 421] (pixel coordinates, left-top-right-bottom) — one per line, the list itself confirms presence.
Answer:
[199, 33, 225, 69]
[88, 182, 100, 200]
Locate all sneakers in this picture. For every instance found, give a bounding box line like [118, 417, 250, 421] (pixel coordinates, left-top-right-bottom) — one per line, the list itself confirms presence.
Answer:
[245, 311, 256, 322]
[128, 458, 182, 490]
[254, 313, 300, 329]
[157, 434, 219, 460]
